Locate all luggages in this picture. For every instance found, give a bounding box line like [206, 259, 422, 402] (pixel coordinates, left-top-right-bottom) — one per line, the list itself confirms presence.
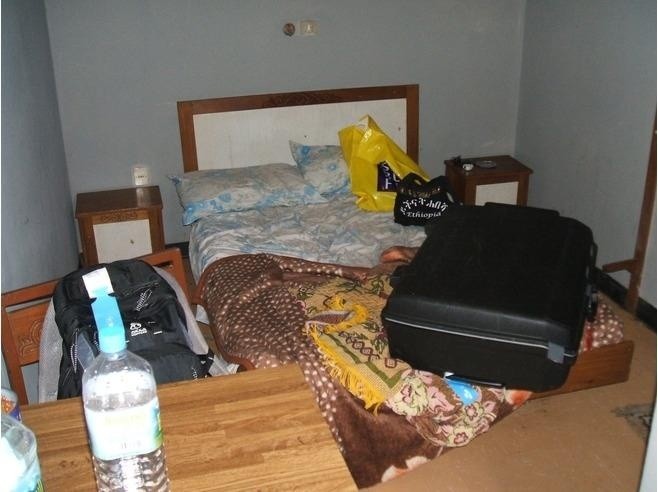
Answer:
[380, 201, 601, 394]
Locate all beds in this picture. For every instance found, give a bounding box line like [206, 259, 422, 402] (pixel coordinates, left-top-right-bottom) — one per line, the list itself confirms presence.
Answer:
[177, 83, 635, 405]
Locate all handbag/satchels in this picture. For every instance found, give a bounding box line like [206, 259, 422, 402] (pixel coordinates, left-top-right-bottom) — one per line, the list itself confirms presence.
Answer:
[339, 112, 435, 212]
[395, 175, 451, 227]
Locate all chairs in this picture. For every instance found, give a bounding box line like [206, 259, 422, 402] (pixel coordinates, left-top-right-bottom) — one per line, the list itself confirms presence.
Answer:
[1, 246, 190, 406]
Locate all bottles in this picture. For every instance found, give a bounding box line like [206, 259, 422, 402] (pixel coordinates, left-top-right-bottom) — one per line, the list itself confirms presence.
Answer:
[81, 328, 172, 492]
[1, 412, 45, 492]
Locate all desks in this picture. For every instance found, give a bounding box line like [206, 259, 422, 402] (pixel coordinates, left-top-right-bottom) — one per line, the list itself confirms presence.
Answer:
[15, 363, 362, 491]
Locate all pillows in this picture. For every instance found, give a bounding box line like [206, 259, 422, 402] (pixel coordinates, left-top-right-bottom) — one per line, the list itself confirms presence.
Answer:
[288, 140, 350, 196]
[167, 163, 329, 227]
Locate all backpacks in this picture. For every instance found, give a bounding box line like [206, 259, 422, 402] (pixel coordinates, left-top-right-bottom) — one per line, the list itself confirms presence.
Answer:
[53, 260, 204, 399]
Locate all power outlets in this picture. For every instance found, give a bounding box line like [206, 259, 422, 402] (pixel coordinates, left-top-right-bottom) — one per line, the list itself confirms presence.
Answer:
[133, 168, 148, 185]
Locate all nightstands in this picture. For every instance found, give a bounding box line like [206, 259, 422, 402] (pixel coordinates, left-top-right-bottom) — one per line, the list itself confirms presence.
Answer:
[444, 154, 533, 207]
[76, 184, 164, 269]
